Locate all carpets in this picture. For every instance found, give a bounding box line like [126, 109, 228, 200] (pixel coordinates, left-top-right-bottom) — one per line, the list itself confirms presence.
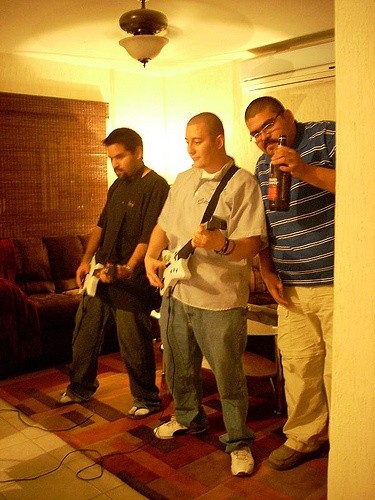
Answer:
[0, 341, 328, 500]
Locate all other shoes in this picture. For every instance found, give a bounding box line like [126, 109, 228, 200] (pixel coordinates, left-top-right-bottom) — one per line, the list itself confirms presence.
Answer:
[57, 392, 74, 405]
[267, 444, 321, 471]
[127, 406, 160, 419]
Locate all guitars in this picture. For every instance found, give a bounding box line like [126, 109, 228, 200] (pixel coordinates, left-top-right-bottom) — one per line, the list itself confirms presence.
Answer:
[159, 217, 223, 297]
[79, 260, 120, 297]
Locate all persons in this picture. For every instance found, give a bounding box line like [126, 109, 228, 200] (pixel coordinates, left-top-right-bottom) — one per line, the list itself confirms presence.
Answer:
[145, 112, 270, 476]
[245, 97, 337, 469]
[48, 127, 171, 418]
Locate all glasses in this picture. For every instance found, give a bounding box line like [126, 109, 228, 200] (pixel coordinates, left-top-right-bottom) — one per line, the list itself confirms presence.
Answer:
[250, 109, 282, 143]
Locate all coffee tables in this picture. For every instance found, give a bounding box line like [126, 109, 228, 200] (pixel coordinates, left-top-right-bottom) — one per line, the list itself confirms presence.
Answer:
[151, 310, 285, 415]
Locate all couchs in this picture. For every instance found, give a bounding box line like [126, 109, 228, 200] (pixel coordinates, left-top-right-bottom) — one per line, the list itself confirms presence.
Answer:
[0, 234, 119, 380]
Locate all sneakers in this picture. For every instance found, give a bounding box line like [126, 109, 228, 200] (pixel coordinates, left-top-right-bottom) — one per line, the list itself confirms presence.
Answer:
[230, 447, 255, 477]
[153, 416, 209, 440]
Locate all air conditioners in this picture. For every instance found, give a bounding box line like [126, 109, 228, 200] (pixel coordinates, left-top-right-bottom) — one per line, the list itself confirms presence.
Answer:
[239, 38, 336, 96]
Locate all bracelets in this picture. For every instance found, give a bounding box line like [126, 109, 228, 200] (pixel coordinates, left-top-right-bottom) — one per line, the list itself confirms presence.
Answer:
[215, 237, 229, 254]
[223, 239, 236, 255]
[123, 264, 134, 279]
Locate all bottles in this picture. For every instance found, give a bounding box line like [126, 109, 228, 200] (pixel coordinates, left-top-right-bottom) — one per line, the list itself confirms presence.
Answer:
[268, 134, 292, 212]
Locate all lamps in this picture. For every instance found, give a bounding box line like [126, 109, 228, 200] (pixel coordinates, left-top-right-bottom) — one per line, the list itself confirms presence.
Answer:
[119, 0, 170, 69]
